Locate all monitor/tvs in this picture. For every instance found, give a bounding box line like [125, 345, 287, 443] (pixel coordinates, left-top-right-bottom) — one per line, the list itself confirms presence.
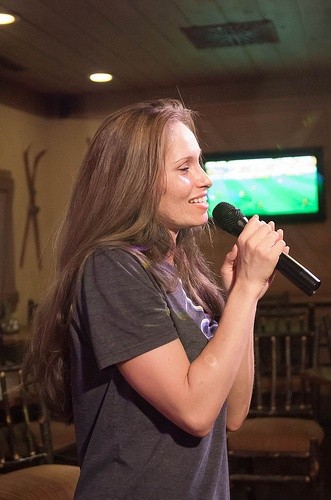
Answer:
[199, 147, 325, 223]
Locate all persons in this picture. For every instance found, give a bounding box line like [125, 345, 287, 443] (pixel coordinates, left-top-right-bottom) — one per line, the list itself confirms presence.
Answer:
[25, 102, 291, 499]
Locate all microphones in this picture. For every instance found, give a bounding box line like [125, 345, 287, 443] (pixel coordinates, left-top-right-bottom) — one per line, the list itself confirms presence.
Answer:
[212, 202, 321, 296]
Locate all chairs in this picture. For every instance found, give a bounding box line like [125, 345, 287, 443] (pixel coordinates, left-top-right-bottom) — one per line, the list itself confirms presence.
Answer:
[1, 363, 82, 500]
[0, 371, 75, 457]
[229, 300, 324, 499]
[304, 302, 331, 422]
